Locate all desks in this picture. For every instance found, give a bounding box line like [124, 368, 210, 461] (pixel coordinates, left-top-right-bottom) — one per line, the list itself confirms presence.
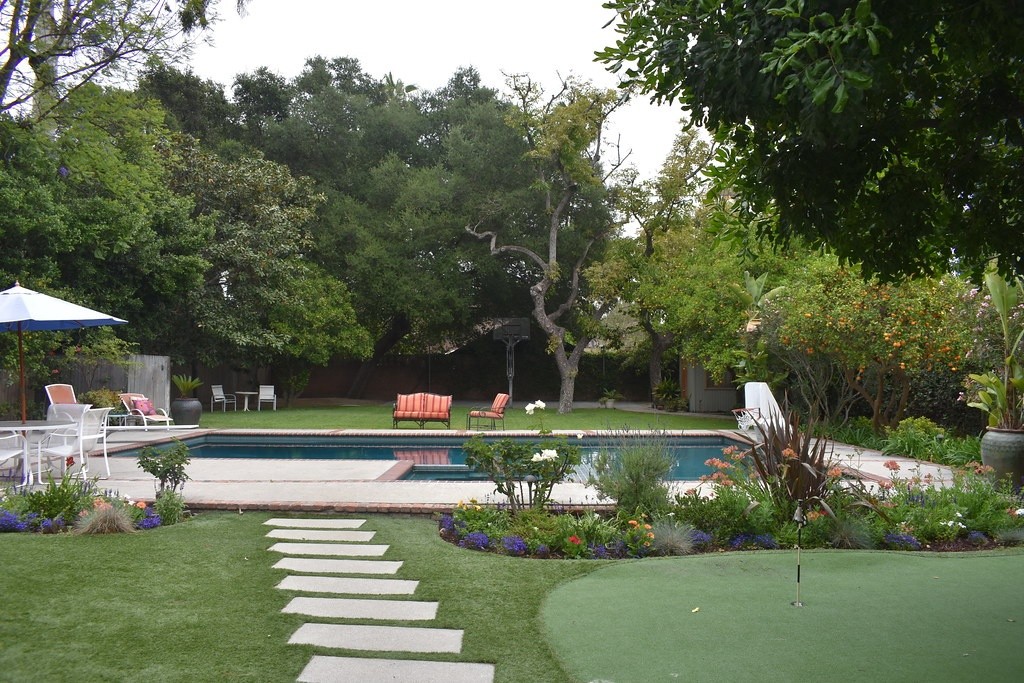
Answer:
[234, 392, 259, 411]
[0, 419, 79, 487]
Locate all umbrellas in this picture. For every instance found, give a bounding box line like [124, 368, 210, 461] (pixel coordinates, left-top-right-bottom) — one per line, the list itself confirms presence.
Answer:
[0, 280, 129, 436]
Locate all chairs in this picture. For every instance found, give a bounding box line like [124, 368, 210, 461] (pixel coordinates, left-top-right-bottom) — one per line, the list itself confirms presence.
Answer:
[258, 384, 277, 411]
[117, 393, 170, 432]
[0, 383, 115, 492]
[209, 384, 237, 413]
[465, 392, 512, 430]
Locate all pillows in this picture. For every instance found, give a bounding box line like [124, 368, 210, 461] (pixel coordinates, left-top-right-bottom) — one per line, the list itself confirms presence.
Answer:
[131, 397, 157, 416]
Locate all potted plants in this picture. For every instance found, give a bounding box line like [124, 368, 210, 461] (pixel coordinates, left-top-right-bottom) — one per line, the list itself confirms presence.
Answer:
[171, 374, 205, 425]
[966, 269, 1024, 494]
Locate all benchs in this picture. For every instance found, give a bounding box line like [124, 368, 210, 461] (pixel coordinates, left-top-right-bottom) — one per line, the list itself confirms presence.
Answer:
[392, 392, 453, 430]
